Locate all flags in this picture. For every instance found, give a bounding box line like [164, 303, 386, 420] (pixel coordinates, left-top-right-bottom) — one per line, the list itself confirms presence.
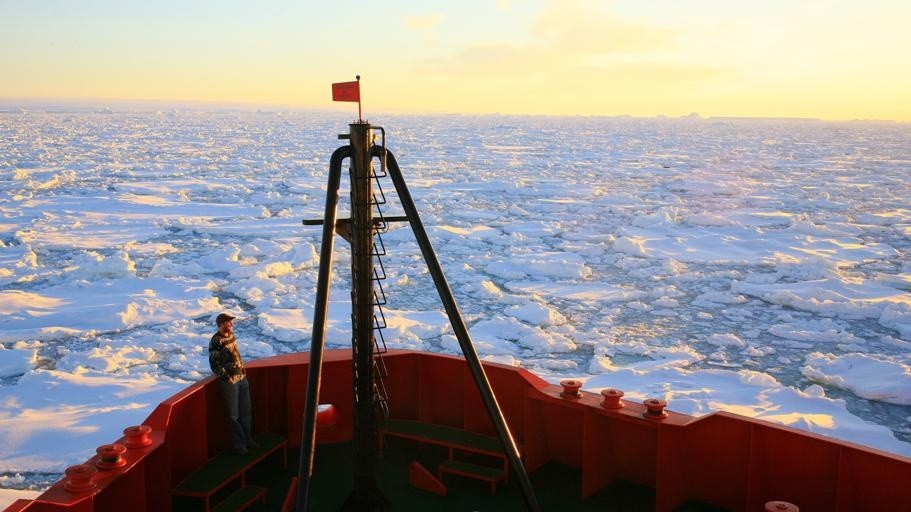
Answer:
[331, 80, 360, 103]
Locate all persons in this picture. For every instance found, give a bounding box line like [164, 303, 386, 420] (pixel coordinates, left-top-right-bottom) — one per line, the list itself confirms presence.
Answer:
[208, 313, 264, 456]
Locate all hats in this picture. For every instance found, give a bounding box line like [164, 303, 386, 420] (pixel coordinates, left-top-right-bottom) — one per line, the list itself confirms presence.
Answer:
[216, 313, 236, 324]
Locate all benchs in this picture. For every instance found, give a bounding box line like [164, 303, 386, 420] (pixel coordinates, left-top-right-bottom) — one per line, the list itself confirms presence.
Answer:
[168, 432, 288, 512]
[375, 420, 510, 482]
[438, 459, 505, 496]
[211, 485, 268, 511]
